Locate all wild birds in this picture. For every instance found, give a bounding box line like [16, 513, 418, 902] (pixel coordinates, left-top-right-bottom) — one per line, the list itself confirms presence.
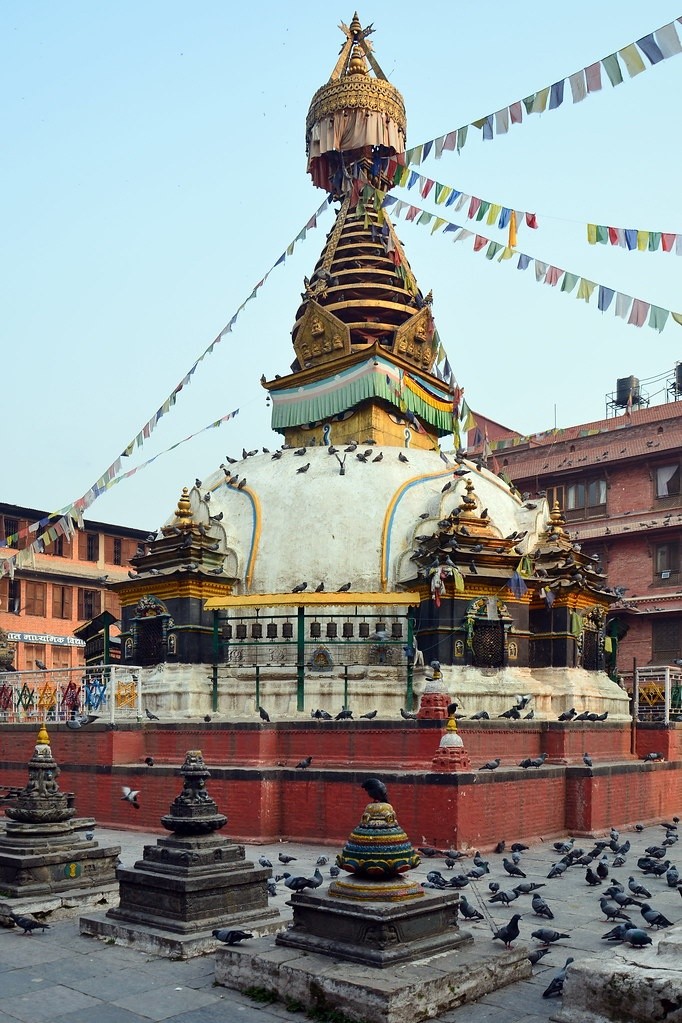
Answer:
[0, 436, 681, 995]
